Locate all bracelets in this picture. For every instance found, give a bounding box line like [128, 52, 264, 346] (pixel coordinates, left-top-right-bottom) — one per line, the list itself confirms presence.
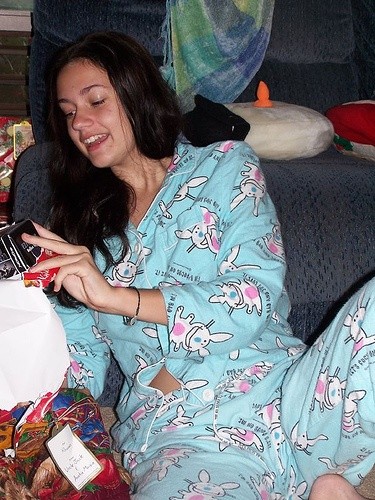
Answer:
[123, 286, 140, 326]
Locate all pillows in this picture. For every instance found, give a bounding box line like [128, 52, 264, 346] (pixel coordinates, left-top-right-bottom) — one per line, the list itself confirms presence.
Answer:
[217, 100, 335, 161]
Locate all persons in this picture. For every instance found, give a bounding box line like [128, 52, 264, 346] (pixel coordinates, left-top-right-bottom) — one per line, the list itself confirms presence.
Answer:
[17, 29, 375, 500]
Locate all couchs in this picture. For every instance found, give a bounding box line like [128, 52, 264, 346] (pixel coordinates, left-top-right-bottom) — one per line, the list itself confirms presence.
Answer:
[12, 139, 375, 500]
